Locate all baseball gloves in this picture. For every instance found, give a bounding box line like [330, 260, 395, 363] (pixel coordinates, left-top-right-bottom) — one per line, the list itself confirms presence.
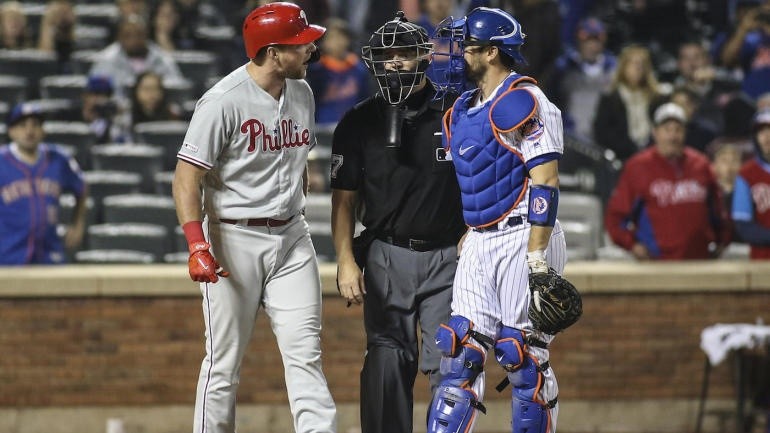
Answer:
[528, 268, 582, 337]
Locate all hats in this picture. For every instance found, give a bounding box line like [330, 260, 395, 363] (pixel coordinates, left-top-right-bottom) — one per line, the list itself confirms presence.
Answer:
[7, 103, 46, 126]
[578, 19, 608, 41]
[85, 76, 114, 97]
[655, 101, 687, 125]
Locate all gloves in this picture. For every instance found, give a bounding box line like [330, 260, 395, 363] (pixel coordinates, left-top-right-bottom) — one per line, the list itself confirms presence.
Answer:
[189, 242, 229, 283]
[528, 251, 552, 312]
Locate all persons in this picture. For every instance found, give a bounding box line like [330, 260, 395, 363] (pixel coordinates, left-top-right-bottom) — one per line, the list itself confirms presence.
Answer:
[0, 0, 770, 266]
[171, 3, 337, 433]
[426, 5, 583, 433]
[328, 0, 472, 433]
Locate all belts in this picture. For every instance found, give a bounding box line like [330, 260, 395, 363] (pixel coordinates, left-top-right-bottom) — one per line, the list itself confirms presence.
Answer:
[474, 216, 524, 232]
[377, 235, 461, 252]
[220, 216, 297, 228]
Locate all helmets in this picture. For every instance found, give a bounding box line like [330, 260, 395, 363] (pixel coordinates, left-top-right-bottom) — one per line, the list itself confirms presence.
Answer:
[361, 11, 435, 105]
[243, 3, 326, 59]
[432, 7, 537, 100]
